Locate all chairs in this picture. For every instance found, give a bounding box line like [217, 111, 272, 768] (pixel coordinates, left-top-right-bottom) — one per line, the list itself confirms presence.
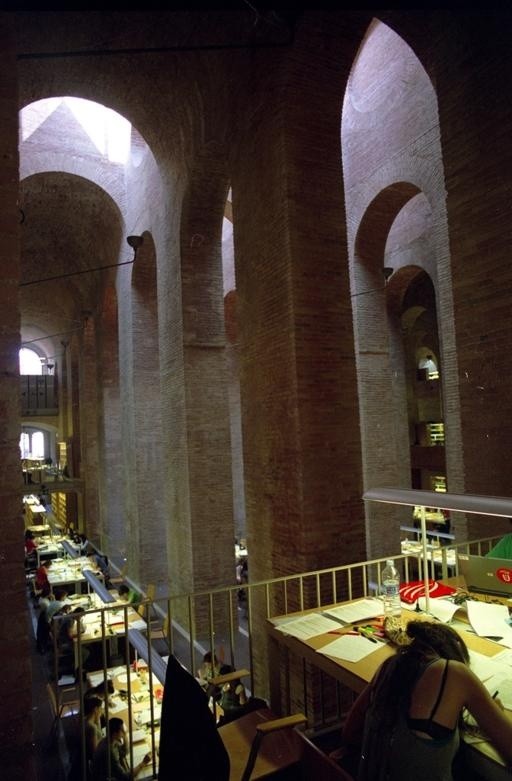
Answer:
[23, 534, 172, 781]
[205, 669, 308, 781]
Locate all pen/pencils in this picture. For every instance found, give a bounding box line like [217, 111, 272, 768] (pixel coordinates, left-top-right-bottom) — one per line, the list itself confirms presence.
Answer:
[492, 691, 498, 699]
[328, 624, 391, 643]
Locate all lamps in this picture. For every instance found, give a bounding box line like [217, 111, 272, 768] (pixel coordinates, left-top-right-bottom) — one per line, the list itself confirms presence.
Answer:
[19, 235, 143, 384]
[362, 486, 512, 615]
[351, 267, 394, 298]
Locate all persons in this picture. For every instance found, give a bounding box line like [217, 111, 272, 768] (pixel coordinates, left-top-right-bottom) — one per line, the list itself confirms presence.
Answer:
[234, 536, 249, 611]
[339, 618, 511, 779]
[35, 584, 89, 672]
[36, 550, 109, 607]
[203, 650, 246, 728]
[119, 585, 146, 614]
[64, 669, 155, 781]
[24, 527, 86, 564]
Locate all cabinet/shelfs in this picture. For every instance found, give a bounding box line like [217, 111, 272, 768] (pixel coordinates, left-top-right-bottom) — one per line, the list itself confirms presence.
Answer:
[23, 490, 78, 531]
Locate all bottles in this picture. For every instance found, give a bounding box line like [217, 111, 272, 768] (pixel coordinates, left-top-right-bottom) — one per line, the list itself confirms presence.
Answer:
[381, 559, 401, 616]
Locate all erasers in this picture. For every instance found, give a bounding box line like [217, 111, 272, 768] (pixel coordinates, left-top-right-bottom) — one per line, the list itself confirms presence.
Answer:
[373, 615, 384, 622]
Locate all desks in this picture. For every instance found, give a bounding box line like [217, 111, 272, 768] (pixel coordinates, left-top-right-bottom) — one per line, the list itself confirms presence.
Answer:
[85, 655, 225, 781]
[265, 576, 512, 781]
[25, 524, 52, 541]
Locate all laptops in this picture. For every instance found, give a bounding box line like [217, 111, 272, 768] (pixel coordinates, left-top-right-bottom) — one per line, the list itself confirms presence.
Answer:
[458, 553, 512, 598]
